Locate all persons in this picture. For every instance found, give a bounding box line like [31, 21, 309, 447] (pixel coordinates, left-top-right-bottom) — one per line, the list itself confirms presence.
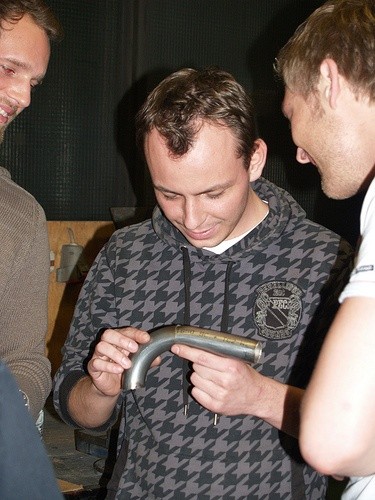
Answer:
[0, 0, 65, 500]
[274, 0, 375, 500]
[53, 69, 357, 500]
